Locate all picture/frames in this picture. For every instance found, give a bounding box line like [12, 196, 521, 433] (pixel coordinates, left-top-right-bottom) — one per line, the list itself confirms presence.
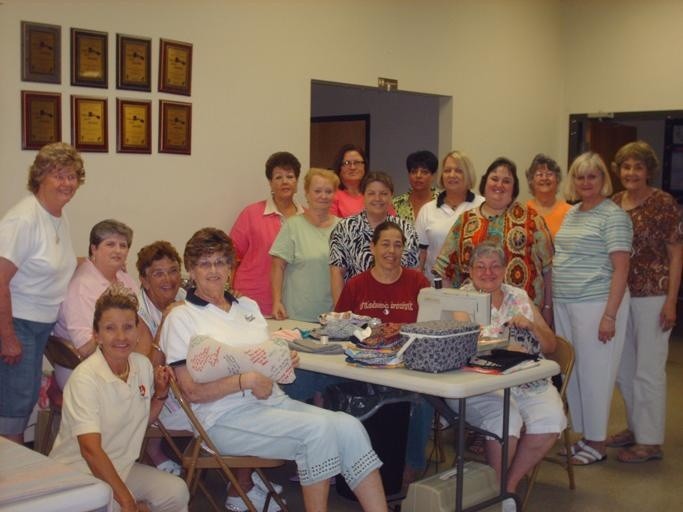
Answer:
[116, 33, 151, 91]
[159, 38, 192, 96]
[70, 94, 108, 152]
[22, 91, 62, 150]
[159, 99, 191, 155]
[116, 97, 152, 154]
[71, 28, 108, 88]
[22, 22, 61, 84]
[312, 114, 370, 173]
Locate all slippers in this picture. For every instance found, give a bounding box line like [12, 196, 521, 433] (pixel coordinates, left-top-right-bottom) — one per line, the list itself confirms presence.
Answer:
[431, 413, 459, 430]
[558, 441, 606, 466]
[140, 454, 186, 478]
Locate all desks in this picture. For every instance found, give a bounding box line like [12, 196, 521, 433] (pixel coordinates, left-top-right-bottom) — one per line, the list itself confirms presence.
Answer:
[264, 316, 561, 512]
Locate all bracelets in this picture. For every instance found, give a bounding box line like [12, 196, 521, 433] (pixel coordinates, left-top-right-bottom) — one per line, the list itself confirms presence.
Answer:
[151, 343, 160, 351]
[154, 393, 168, 400]
[604, 314, 614, 321]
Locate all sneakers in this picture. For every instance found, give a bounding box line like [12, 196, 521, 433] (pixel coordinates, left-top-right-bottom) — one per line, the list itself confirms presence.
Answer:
[225, 471, 287, 511]
[617, 444, 663, 464]
[469, 432, 485, 454]
[605, 429, 635, 448]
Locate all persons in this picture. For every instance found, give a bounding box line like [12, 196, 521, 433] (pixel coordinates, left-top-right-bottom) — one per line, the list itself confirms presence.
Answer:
[135, 240, 286, 512]
[609, 140, 683, 463]
[46, 295, 190, 512]
[443, 242, 568, 512]
[227, 144, 574, 495]
[53, 220, 187, 479]
[0, 142, 86, 445]
[158, 227, 389, 512]
[550, 151, 634, 467]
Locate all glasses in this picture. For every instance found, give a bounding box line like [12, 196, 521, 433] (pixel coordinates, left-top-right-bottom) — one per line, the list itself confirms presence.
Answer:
[192, 259, 229, 271]
[471, 262, 504, 273]
[146, 267, 181, 279]
[47, 171, 80, 183]
[341, 159, 364, 168]
[532, 170, 557, 180]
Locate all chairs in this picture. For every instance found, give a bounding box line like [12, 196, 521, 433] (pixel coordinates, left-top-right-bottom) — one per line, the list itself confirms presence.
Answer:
[137, 414, 228, 511]
[42, 336, 86, 457]
[169, 375, 288, 511]
[520, 336, 576, 512]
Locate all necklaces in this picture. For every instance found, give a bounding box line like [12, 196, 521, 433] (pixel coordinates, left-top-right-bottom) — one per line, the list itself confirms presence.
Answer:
[39, 199, 63, 243]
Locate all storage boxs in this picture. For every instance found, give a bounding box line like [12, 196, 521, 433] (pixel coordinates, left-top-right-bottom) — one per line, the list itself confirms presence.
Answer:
[400, 321, 480, 372]
